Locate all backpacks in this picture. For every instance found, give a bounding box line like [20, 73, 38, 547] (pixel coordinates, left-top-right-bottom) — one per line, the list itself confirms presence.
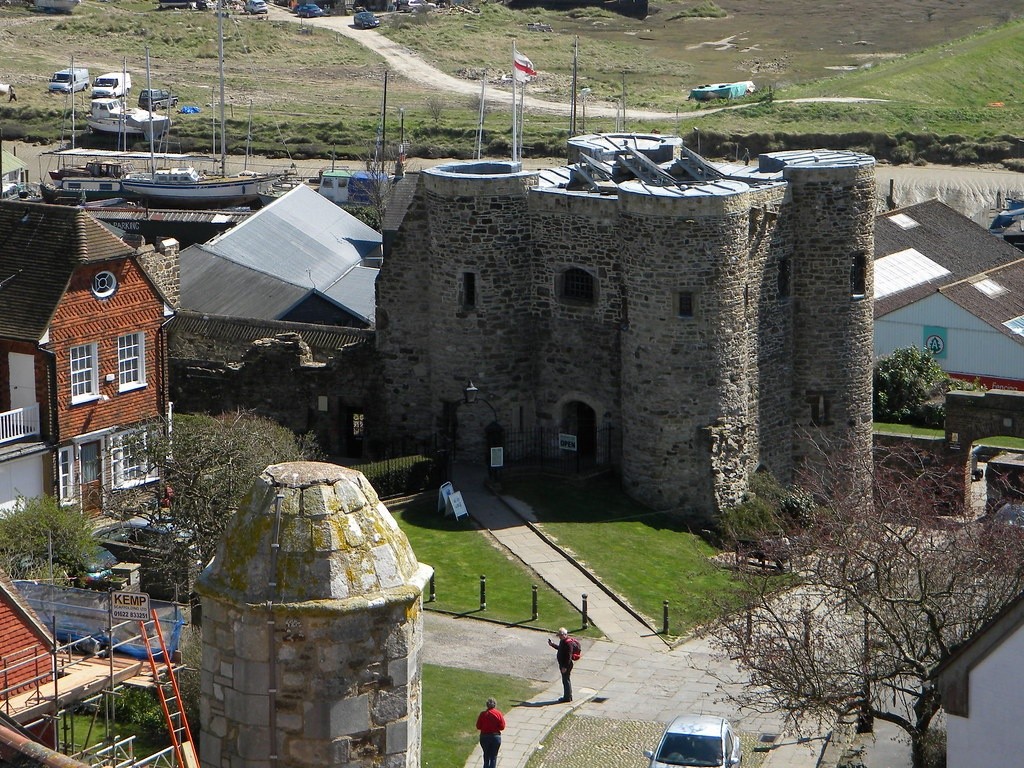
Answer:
[565, 638, 581, 660]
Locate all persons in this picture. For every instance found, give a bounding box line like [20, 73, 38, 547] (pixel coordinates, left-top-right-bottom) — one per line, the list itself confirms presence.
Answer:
[8, 85, 17, 102]
[549, 627, 574, 702]
[476, 698, 505, 768]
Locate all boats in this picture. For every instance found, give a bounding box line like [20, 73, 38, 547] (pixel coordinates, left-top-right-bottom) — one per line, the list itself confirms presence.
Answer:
[85, 98, 172, 137]
[34, 0, 81, 13]
[690, 82, 746, 103]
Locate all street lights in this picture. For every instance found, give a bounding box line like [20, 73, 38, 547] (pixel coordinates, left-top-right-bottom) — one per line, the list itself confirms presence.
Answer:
[693, 127, 700, 155]
[464, 380, 498, 445]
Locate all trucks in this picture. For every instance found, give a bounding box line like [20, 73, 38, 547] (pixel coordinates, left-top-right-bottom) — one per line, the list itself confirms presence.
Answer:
[48, 68, 89, 94]
[92, 73, 131, 99]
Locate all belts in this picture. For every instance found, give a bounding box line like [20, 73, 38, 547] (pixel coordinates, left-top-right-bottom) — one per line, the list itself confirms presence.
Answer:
[481, 731, 500, 734]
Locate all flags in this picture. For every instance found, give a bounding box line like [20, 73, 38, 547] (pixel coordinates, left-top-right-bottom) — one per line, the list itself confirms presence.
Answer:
[514, 52, 537, 82]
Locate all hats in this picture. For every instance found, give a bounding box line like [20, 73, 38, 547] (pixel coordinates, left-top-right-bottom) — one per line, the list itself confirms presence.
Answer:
[556, 627, 568, 636]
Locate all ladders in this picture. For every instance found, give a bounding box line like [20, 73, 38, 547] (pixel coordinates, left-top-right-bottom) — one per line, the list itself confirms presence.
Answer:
[138, 609, 202, 768]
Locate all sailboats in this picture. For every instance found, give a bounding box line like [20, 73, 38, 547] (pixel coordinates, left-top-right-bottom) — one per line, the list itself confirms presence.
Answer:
[48, 54, 136, 187]
[256, 71, 394, 206]
[121, 0, 298, 207]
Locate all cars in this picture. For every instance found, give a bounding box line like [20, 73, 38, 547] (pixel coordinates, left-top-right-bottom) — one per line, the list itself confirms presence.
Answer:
[399, 0, 436, 13]
[138, 89, 179, 111]
[297, 4, 323, 18]
[643, 714, 743, 768]
[91, 518, 199, 554]
[244, 0, 268, 15]
[979, 498, 1024, 578]
[354, 12, 380, 30]
[41, 542, 117, 594]
